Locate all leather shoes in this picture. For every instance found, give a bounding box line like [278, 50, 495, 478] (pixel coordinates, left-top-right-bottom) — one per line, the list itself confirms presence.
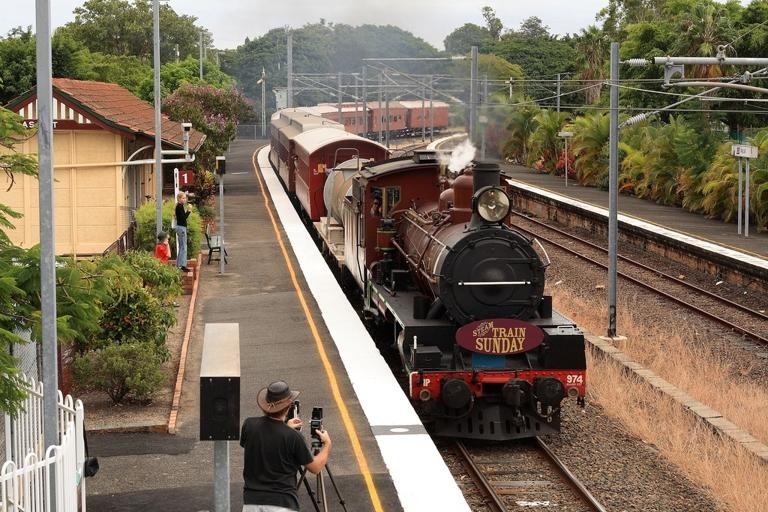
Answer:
[181, 266, 191, 272]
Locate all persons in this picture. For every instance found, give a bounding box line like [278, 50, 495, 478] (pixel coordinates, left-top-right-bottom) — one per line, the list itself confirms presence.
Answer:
[155, 231, 171, 264]
[240, 380, 331, 512]
[175, 192, 192, 272]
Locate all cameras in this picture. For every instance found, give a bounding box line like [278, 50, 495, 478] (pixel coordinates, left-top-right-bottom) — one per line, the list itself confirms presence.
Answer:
[307, 407, 324, 439]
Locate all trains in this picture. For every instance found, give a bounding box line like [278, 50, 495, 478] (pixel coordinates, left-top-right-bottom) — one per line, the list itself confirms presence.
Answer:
[265, 96, 588, 446]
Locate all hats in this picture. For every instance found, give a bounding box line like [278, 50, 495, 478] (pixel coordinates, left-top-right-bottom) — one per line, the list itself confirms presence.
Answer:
[257, 380, 300, 413]
[158, 230, 168, 240]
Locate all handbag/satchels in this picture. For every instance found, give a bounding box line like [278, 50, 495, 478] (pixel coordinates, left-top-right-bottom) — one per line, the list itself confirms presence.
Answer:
[172, 218, 177, 230]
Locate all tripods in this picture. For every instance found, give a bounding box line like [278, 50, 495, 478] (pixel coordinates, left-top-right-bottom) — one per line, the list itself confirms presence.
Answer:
[299, 444, 350, 511]
[294, 464, 319, 512]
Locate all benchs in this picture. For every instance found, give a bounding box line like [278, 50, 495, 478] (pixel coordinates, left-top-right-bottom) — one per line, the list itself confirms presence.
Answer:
[204, 223, 228, 264]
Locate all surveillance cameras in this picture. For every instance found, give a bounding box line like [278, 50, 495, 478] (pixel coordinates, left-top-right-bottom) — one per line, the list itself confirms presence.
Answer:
[181, 122, 192, 132]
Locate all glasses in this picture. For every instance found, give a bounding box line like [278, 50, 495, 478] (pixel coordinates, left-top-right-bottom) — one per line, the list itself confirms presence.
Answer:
[374, 202, 378, 205]
